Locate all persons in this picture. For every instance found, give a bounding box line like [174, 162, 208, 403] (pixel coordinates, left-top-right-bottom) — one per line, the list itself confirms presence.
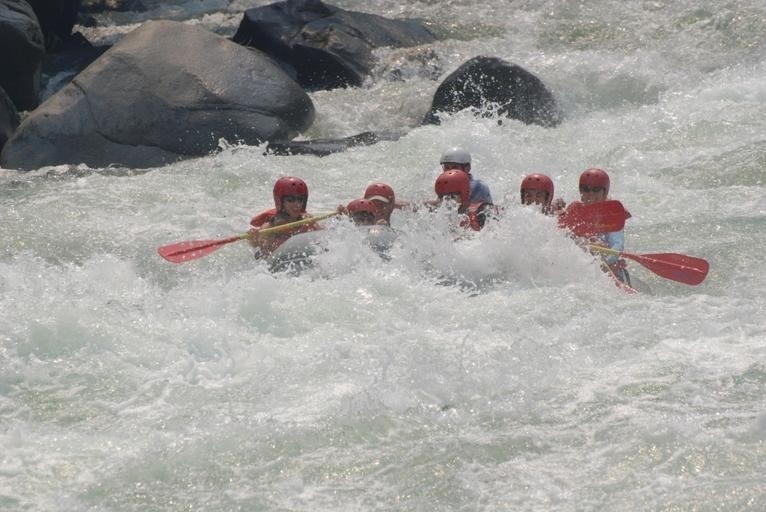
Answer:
[438, 146, 491, 203]
[247, 176, 326, 260]
[521, 168, 632, 287]
[336, 183, 395, 225]
[413, 170, 485, 231]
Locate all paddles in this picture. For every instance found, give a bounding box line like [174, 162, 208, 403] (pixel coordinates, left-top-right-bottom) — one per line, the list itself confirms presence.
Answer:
[557, 201, 625, 235]
[158, 211, 341, 263]
[580, 245, 708, 286]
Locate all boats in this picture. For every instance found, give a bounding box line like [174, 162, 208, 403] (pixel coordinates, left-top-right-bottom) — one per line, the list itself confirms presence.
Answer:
[255, 218, 655, 301]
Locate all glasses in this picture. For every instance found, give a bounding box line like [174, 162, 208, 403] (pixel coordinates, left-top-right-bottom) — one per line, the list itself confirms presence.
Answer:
[582, 185, 602, 192]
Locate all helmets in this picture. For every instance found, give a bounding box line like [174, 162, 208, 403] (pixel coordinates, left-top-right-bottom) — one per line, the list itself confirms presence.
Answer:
[364, 183, 395, 214]
[579, 168, 610, 202]
[440, 147, 471, 170]
[347, 199, 378, 215]
[435, 169, 469, 207]
[274, 176, 308, 212]
[521, 173, 554, 213]
